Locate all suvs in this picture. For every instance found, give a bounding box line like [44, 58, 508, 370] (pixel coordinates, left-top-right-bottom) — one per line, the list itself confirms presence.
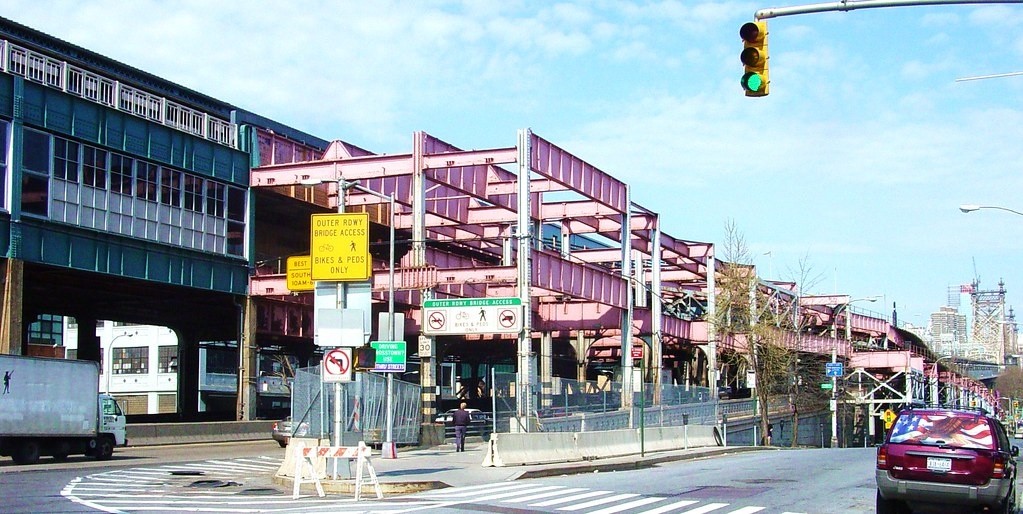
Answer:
[875, 403, 1019, 514]
[718, 378, 751, 400]
[537, 405, 579, 430]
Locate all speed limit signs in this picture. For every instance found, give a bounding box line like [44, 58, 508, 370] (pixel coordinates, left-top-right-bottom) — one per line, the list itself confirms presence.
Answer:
[419, 336, 432, 358]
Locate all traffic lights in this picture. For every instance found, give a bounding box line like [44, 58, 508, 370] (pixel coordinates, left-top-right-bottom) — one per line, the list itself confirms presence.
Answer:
[740, 20, 769, 98]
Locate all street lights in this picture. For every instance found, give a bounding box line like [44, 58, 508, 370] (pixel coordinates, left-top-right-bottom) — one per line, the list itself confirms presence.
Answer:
[829, 297, 878, 448]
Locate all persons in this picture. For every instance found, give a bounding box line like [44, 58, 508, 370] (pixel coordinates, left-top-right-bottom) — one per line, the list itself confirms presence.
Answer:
[767, 419, 774, 446]
[451, 403, 471, 452]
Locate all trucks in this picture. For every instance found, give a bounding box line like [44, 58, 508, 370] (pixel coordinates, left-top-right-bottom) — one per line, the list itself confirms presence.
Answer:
[0, 352, 131, 466]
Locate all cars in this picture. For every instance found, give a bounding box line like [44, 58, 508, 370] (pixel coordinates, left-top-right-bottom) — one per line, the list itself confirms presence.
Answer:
[434, 408, 489, 437]
[272, 416, 312, 449]
